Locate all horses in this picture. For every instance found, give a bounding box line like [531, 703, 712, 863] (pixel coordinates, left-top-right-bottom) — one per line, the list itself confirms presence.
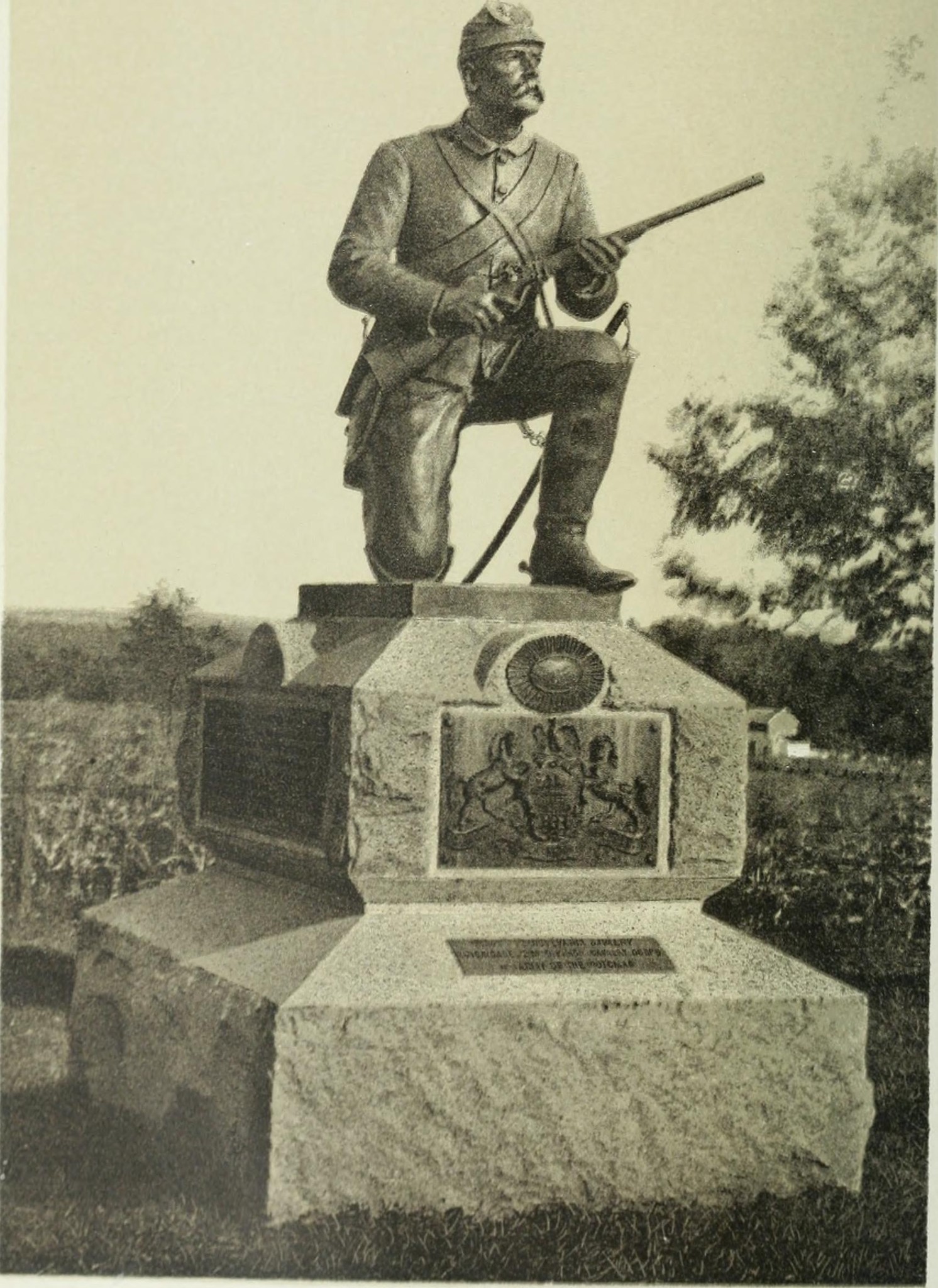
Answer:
[581, 734, 650, 836]
[446, 732, 528, 830]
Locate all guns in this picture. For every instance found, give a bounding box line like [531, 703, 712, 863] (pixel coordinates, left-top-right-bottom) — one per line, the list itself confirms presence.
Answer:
[356, 171, 771, 393]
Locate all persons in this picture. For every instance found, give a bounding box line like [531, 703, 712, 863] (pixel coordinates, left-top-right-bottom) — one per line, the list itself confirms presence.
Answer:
[329, 5, 637, 596]
